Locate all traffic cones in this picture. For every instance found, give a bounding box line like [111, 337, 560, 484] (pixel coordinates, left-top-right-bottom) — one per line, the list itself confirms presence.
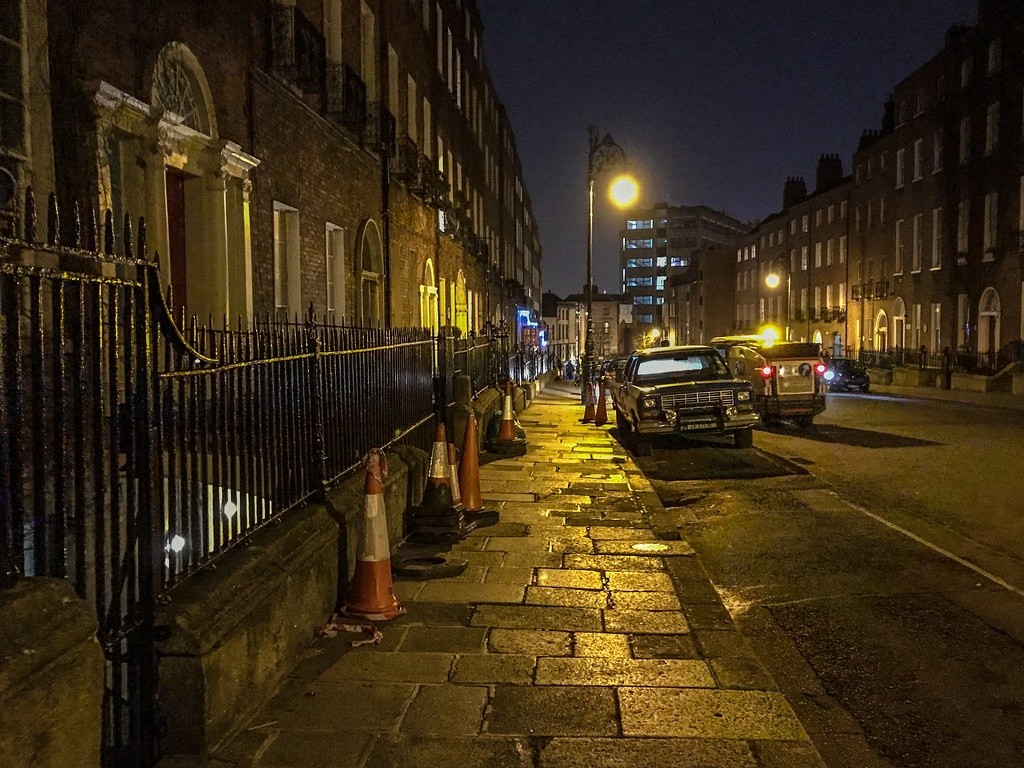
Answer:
[492, 380, 523, 443]
[448, 443, 466, 513]
[589, 382, 613, 424]
[416, 421, 466, 518]
[577, 381, 596, 422]
[338, 451, 407, 621]
[458, 411, 485, 513]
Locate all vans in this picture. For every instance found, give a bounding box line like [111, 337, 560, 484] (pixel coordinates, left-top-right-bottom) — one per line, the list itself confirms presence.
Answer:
[710, 335, 827, 427]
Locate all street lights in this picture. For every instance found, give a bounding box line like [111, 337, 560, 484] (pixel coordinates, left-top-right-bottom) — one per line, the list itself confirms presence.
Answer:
[765, 250, 792, 342]
[580, 125, 641, 406]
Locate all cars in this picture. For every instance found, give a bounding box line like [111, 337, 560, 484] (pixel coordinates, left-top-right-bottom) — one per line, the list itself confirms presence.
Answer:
[595, 358, 628, 389]
[608, 344, 762, 457]
[823, 356, 870, 393]
